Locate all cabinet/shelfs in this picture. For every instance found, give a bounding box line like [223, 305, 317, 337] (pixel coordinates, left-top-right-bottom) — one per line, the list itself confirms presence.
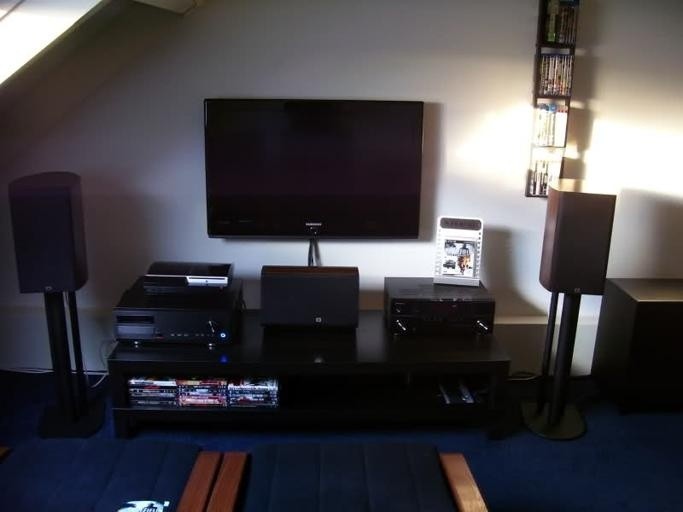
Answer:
[106, 308, 511, 442]
[590, 277, 682, 414]
[525, 0, 579, 197]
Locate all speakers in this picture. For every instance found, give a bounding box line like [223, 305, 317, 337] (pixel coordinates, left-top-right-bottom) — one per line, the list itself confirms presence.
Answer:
[7, 171, 88, 294]
[540, 180, 616, 294]
[260, 267, 358, 330]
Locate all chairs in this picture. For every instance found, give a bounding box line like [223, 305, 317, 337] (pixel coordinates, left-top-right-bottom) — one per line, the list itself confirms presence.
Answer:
[0, 446, 221, 512]
[206, 452, 489, 512]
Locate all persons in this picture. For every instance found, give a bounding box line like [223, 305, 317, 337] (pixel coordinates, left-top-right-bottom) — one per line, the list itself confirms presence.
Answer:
[458, 243, 470, 275]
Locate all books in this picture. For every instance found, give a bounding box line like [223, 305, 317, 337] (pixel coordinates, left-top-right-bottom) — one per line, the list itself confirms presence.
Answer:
[127, 375, 279, 407]
[528, 0, 580, 196]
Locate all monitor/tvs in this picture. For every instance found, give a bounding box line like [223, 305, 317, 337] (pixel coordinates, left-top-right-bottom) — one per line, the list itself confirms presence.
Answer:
[202, 97, 424, 239]
